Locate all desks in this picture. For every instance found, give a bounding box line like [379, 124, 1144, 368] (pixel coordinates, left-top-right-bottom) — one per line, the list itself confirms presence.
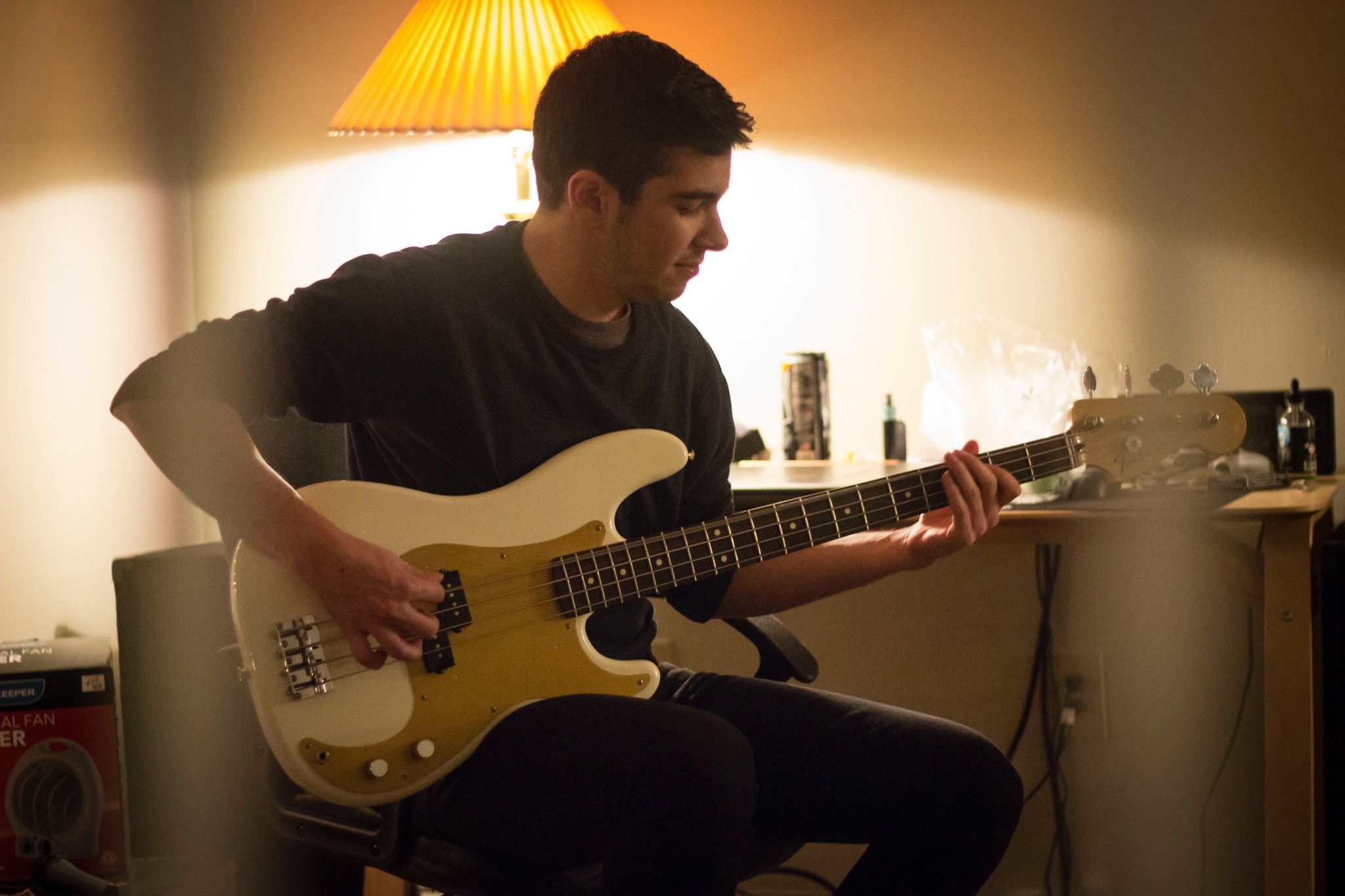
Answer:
[723, 452, 1345, 896]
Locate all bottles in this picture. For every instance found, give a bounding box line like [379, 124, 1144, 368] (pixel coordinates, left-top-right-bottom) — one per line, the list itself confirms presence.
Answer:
[1275, 378, 1319, 480]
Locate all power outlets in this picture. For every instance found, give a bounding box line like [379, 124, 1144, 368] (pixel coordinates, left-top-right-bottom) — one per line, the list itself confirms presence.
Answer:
[1052, 650, 1109, 736]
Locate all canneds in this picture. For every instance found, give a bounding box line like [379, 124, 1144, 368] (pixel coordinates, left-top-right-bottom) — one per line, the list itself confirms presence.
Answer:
[781, 350, 831, 462]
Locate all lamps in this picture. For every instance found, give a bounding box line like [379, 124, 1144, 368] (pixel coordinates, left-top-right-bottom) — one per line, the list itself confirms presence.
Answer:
[328, 0, 625, 223]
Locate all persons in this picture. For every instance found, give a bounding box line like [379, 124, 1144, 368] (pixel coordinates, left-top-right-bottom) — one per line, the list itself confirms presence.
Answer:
[111, 31, 1023, 896]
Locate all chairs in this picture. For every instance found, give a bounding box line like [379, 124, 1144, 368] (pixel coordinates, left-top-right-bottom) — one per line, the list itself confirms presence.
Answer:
[219, 405, 808, 896]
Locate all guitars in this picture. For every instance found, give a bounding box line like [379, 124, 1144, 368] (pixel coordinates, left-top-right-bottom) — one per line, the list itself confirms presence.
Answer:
[223, 355, 1250, 810]
